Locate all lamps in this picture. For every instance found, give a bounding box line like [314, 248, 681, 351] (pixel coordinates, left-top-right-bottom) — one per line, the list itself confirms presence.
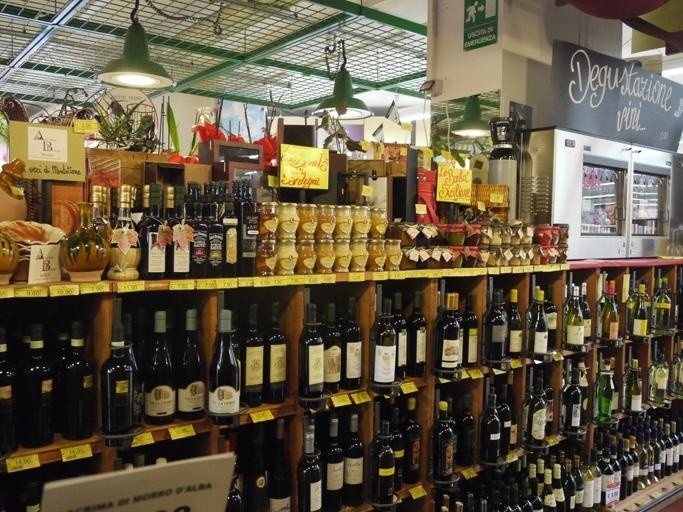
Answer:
[97, 0, 172, 89]
[313, 41, 372, 121]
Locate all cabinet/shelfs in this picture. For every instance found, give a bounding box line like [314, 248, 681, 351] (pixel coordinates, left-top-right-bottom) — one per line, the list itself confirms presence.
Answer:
[516, 126, 682, 262]
[0, 260, 683, 512]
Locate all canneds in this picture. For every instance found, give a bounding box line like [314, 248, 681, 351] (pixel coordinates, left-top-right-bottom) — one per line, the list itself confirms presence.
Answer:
[298, 240, 317, 275]
[278, 202, 301, 239]
[384, 221, 569, 271]
[278, 239, 297, 275]
[369, 239, 387, 272]
[298, 202, 387, 239]
[257, 202, 279, 240]
[336, 239, 352, 272]
[317, 239, 335, 274]
[352, 238, 369, 271]
[257, 239, 277, 278]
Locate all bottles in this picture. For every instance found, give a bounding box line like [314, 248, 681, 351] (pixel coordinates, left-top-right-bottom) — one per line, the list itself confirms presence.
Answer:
[349, 170, 363, 204]
[90, 176, 257, 279]
[337, 172, 349, 204]
[0, 277, 683, 512]
